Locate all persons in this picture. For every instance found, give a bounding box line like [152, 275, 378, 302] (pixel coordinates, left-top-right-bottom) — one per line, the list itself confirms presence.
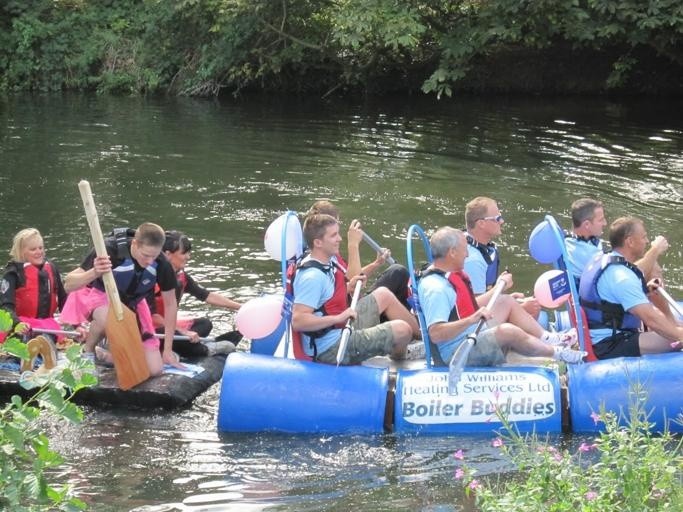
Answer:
[307, 199, 411, 309]
[565, 198, 670, 292]
[290, 213, 422, 367]
[146, 230, 242, 358]
[578, 215, 683, 361]
[0, 227, 89, 364]
[418, 225, 587, 368]
[63, 222, 188, 377]
[463, 195, 542, 330]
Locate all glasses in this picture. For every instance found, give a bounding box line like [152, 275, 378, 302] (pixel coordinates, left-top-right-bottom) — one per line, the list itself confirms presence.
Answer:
[474, 215, 502, 222]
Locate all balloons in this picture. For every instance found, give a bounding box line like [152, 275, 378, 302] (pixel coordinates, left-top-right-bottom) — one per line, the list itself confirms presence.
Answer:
[533, 268, 569, 308]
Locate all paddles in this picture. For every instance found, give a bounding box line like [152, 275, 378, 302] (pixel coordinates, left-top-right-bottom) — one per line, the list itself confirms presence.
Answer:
[78, 180, 150, 391]
[449, 270, 509, 391]
[336, 273, 363, 366]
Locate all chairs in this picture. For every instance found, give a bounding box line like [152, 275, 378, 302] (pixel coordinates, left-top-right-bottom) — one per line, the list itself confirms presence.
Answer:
[406, 277, 443, 366]
[568, 295, 597, 364]
[285, 264, 314, 359]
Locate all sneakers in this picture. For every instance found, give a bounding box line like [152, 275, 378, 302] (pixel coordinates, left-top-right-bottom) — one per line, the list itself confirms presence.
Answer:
[206, 340, 236, 356]
[554, 347, 589, 363]
[554, 328, 578, 347]
[80, 344, 96, 376]
[405, 343, 425, 360]
[20, 339, 40, 373]
[36, 336, 55, 369]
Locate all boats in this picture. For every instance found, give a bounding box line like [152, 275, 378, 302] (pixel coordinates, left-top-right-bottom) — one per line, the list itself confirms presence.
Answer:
[44, 330, 243, 412]
[216, 209, 681, 442]
